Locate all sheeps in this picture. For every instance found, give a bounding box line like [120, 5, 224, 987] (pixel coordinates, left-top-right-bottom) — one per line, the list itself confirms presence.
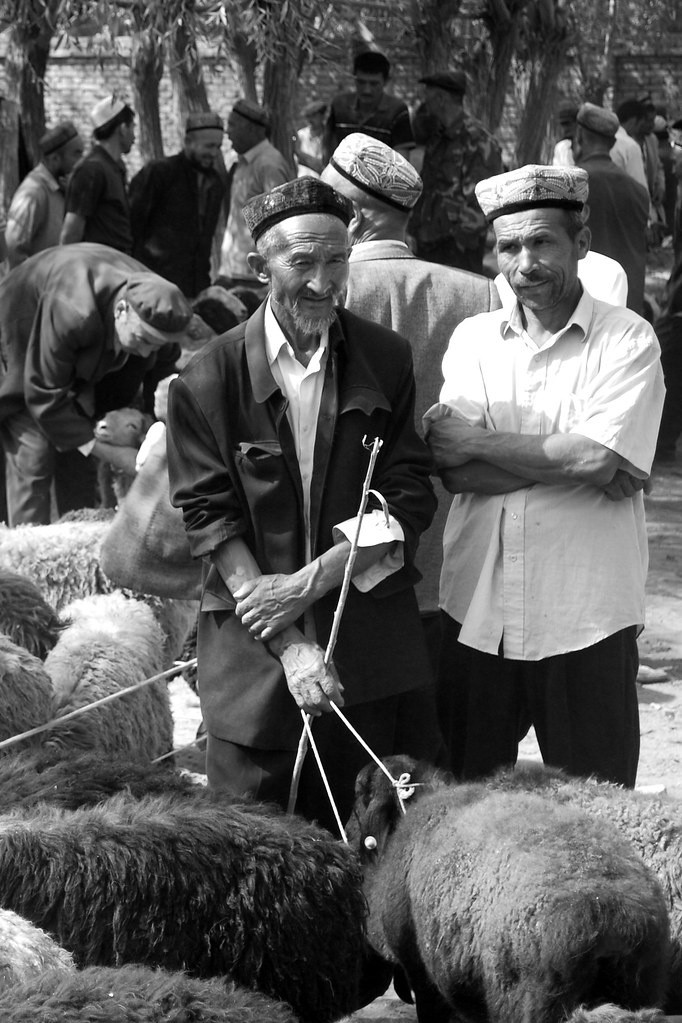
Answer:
[1, 963, 298, 1023]
[93, 407, 154, 507]
[0, 509, 281, 818]
[486, 761, 682, 1016]
[0, 791, 392, 1023]
[343, 754, 671, 1023]
[0, 907, 76, 989]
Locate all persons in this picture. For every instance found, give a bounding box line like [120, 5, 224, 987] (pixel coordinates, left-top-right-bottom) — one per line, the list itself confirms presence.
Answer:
[4, 100, 293, 315]
[153, 133, 503, 761]
[559, 98, 681, 332]
[0, 244, 191, 529]
[420, 163, 668, 789]
[167, 175, 446, 836]
[295, 54, 502, 275]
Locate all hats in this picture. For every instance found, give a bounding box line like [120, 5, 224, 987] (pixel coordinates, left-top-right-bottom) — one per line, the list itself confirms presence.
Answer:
[651, 115, 667, 132]
[559, 110, 577, 123]
[302, 100, 328, 118]
[328, 133, 423, 213]
[233, 98, 270, 128]
[240, 175, 355, 246]
[578, 103, 620, 139]
[38, 122, 78, 155]
[123, 271, 195, 342]
[636, 92, 652, 106]
[418, 72, 467, 99]
[186, 112, 223, 134]
[89, 97, 129, 130]
[475, 165, 589, 221]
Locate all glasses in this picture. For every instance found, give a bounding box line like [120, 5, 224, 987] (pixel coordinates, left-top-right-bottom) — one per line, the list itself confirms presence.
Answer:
[560, 119, 576, 128]
